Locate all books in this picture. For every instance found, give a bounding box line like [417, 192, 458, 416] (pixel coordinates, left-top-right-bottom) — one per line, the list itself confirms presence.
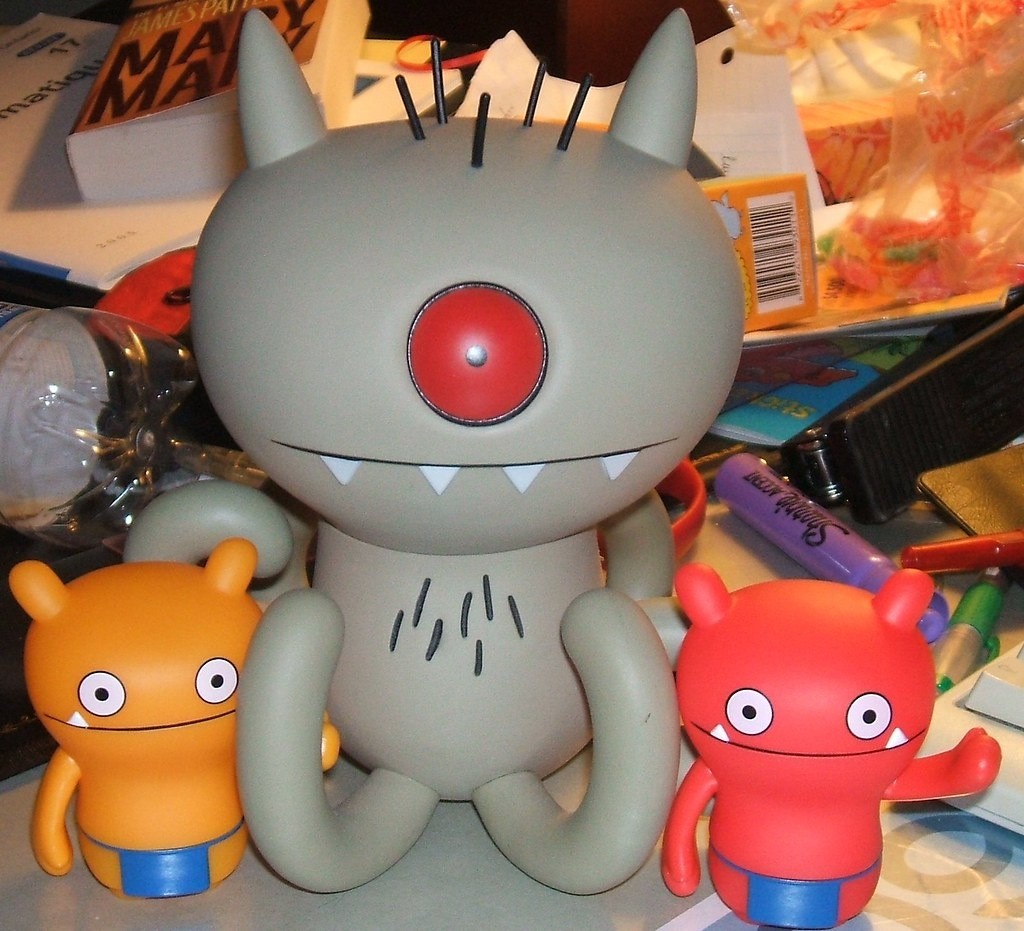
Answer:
[64, 0, 370, 211]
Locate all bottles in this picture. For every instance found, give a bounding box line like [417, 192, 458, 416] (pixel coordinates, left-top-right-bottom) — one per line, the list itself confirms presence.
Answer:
[0, 300, 228, 549]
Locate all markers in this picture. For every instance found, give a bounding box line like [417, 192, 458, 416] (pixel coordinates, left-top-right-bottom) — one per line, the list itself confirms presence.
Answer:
[934, 568, 1012, 691]
[902, 530, 1024, 574]
[713, 454, 949, 646]
